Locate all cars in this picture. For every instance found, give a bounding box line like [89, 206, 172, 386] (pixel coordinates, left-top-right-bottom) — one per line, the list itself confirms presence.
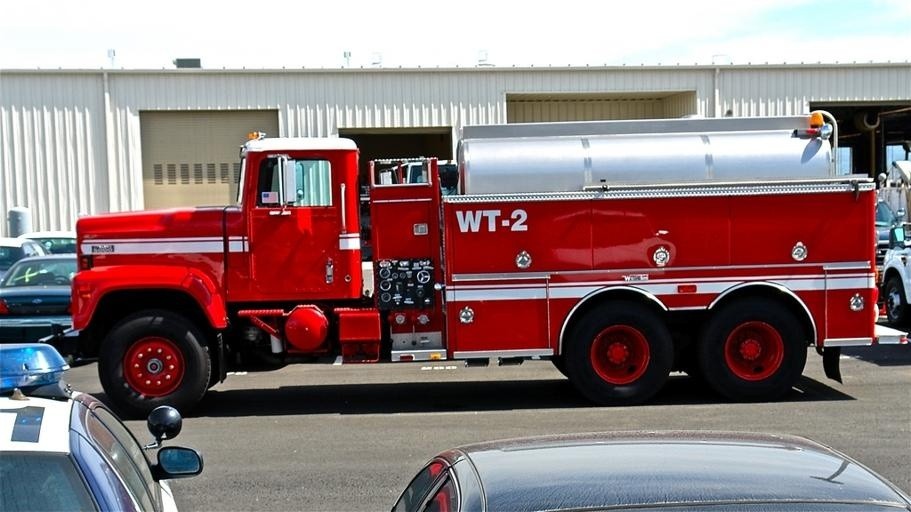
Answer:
[391, 429, 911, 512]
[875, 199, 911, 325]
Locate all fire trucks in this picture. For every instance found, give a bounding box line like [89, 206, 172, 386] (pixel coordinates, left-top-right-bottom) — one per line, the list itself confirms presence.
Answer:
[67, 110, 879, 417]
